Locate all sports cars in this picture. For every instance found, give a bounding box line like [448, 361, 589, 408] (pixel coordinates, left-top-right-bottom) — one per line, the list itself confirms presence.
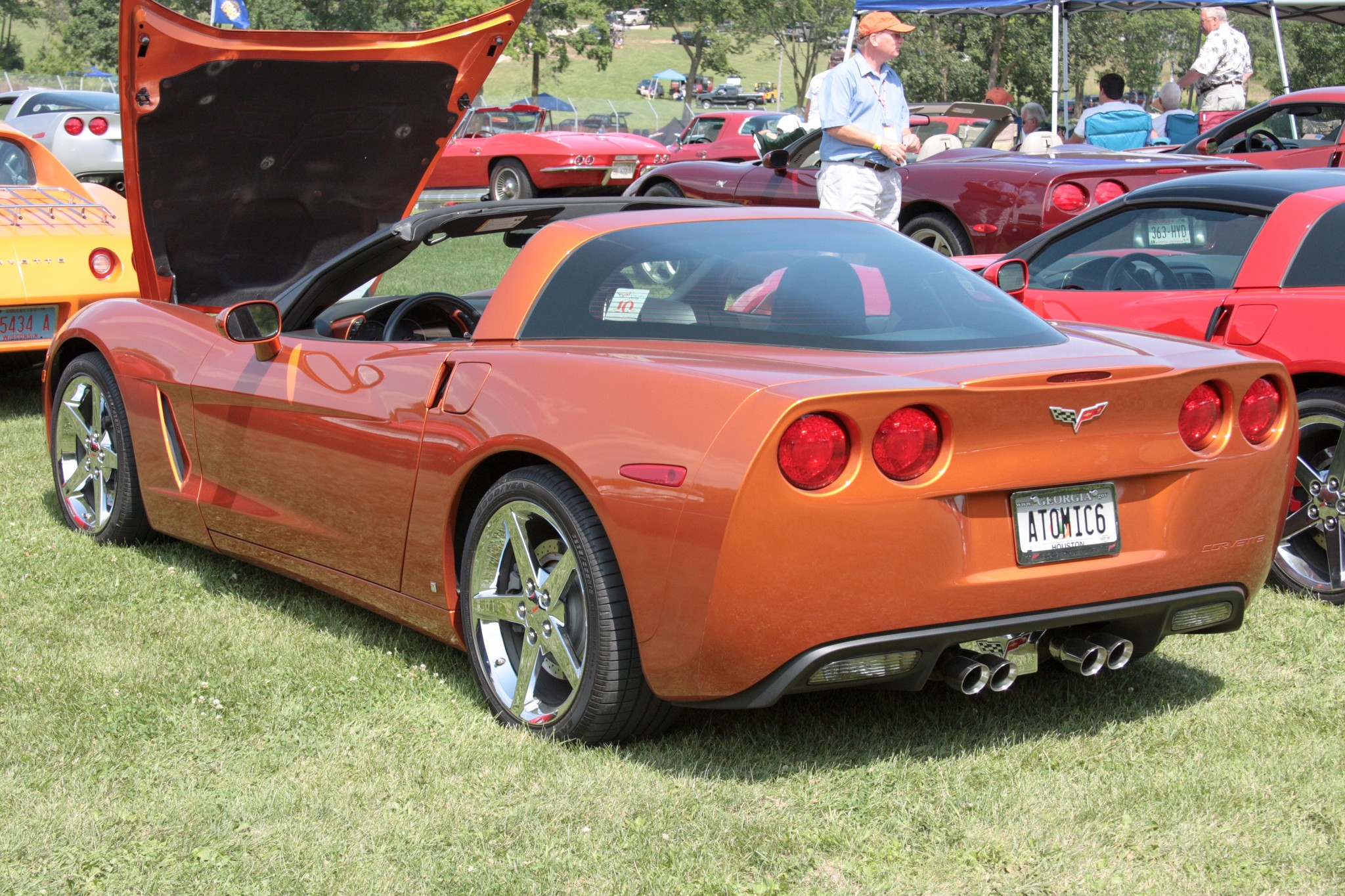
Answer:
[39, 0, 1303, 754]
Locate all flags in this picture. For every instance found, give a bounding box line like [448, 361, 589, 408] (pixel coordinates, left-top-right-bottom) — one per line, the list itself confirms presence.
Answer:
[211, 0, 251, 30]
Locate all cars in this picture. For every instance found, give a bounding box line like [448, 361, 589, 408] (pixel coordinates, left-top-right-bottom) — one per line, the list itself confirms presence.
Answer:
[1116, 85, 1345, 174]
[1058, 88, 1160, 114]
[410, 102, 673, 212]
[0, 88, 127, 195]
[637, 80, 661, 97]
[663, 100, 1024, 217]
[620, 113, 1266, 271]
[946, 164, 1345, 607]
[672, 31, 714, 46]
[780, 21, 857, 49]
[606, 8, 650, 26]
[0, 118, 145, 379]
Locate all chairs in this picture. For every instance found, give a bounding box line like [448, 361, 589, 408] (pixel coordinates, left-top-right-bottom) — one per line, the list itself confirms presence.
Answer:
[1164, 113, 1200, 145]
[915, 133, 962, 162]
[1018, 130, 1063, 153]
[1085, 109, 1171, 151]
[1199, 109, 1248, 139]
[702, 123, 723, 143]
[770, 253, 871, 336]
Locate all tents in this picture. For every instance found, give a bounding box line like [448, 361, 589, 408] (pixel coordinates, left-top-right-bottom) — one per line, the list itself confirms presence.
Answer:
[645, 69, 687, 100]
[842, 0, 1345, 159]
[68, 66, 113, 78]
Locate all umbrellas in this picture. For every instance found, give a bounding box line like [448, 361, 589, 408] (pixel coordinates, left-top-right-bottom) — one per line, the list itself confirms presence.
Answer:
[511, 93, 577, 112]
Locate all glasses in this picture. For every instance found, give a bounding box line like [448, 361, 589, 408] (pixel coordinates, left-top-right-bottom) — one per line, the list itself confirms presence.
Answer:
[881, 30, 901, 40]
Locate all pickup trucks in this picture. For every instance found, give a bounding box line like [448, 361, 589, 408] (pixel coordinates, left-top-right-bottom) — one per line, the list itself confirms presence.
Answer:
[693, 86, 766, 109]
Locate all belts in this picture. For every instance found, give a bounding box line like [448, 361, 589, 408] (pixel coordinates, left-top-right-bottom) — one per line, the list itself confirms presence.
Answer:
[849, 157, 890, 172]
[1198, 81, 1242, 97]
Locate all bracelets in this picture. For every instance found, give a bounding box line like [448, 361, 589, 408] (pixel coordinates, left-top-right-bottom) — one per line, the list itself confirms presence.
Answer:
[872, 135, 882, 150]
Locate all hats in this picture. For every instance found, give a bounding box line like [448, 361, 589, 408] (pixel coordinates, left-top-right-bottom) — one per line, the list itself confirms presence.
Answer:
[858, 11, 916, 41]
[984, 87, 1014, 107]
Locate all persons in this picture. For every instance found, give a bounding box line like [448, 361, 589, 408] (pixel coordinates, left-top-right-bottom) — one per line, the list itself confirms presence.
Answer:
[640, 83, 698, 101]
[814, 10, 922, 234]
[984, 87, 1064, 155]
[1057, 73, 1152, 149]
[1151, 82, 1195, 146]
[598, 125, 605, 133]
[1158, 6, 1254, 111]
[803, 51, 847, 123]
[721, 89, 727, 95]
